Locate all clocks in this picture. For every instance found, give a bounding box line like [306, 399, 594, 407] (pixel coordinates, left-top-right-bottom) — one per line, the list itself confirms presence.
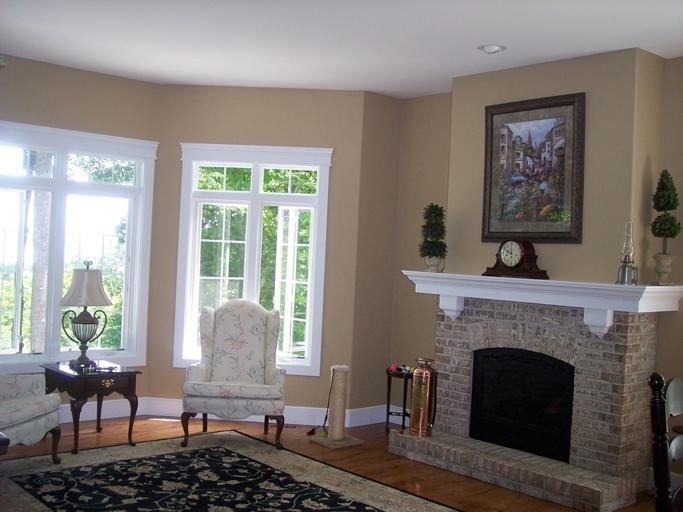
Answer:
[499, 239, 522, 267]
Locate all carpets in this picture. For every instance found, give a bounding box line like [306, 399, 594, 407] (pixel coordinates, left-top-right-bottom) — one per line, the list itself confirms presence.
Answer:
[0, 429, 463, 511]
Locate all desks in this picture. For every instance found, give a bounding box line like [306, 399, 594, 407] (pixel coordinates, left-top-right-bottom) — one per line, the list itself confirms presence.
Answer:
[38, 359, 144, 454]
[385, 366, 437, 433]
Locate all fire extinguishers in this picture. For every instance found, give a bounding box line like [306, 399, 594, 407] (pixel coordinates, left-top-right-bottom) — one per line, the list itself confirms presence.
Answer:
[409, 356, 439, 437]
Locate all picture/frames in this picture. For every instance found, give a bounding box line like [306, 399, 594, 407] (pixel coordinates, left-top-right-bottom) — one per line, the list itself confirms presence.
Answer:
[480, 92, 584, 244]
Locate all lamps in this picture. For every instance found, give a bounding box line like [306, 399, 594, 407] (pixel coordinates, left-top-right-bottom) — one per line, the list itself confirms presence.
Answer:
[57, 259, 114, 369]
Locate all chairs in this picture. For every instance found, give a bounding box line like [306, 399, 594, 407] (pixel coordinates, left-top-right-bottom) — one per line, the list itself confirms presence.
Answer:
[177, 298, 289, 450]
[0, 371, 61, 465]
[646, 370, 683, 511]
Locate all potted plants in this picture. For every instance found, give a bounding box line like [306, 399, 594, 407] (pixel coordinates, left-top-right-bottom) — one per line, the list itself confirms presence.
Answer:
[418, 201, 449, 273]
[650, 167, 683, 286]
